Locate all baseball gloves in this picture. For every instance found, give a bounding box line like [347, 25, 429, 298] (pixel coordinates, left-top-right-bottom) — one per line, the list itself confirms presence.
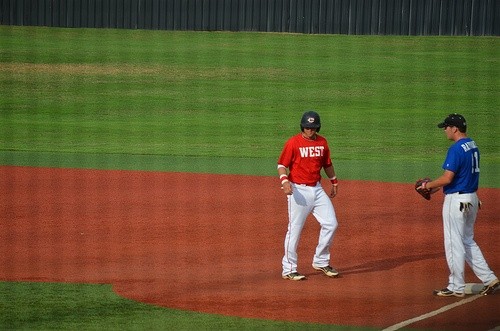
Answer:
[415, 178, 440, 200]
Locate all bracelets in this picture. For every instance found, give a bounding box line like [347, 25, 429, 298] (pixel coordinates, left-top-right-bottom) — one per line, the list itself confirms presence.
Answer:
[328, 176, 338, 186]
[279, 174, 288, 185]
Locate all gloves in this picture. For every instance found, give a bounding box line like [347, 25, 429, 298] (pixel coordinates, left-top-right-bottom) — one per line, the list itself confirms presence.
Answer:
[460, 202, 473, 213]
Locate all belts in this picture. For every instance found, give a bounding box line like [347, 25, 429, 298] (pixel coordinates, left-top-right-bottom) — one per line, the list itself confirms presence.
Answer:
[296, 183, 317, 186]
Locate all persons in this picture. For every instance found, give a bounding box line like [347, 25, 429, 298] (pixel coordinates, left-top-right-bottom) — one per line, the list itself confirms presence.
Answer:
[277, 110, 340, 280]
[415, 113, 500, 297]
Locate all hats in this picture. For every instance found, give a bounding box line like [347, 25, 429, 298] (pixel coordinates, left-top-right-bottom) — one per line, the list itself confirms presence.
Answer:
[437, 113, 466, 129]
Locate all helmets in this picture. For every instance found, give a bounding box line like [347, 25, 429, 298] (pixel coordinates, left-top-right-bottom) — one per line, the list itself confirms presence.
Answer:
[300, 111, 321, 132]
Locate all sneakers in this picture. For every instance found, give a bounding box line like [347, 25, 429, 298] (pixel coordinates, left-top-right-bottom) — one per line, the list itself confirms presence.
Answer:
[282, 272, 305, 281]
[312, 264, 338, 277]
[479, 279, 500, 296]
[433, 288, 465, 297]
